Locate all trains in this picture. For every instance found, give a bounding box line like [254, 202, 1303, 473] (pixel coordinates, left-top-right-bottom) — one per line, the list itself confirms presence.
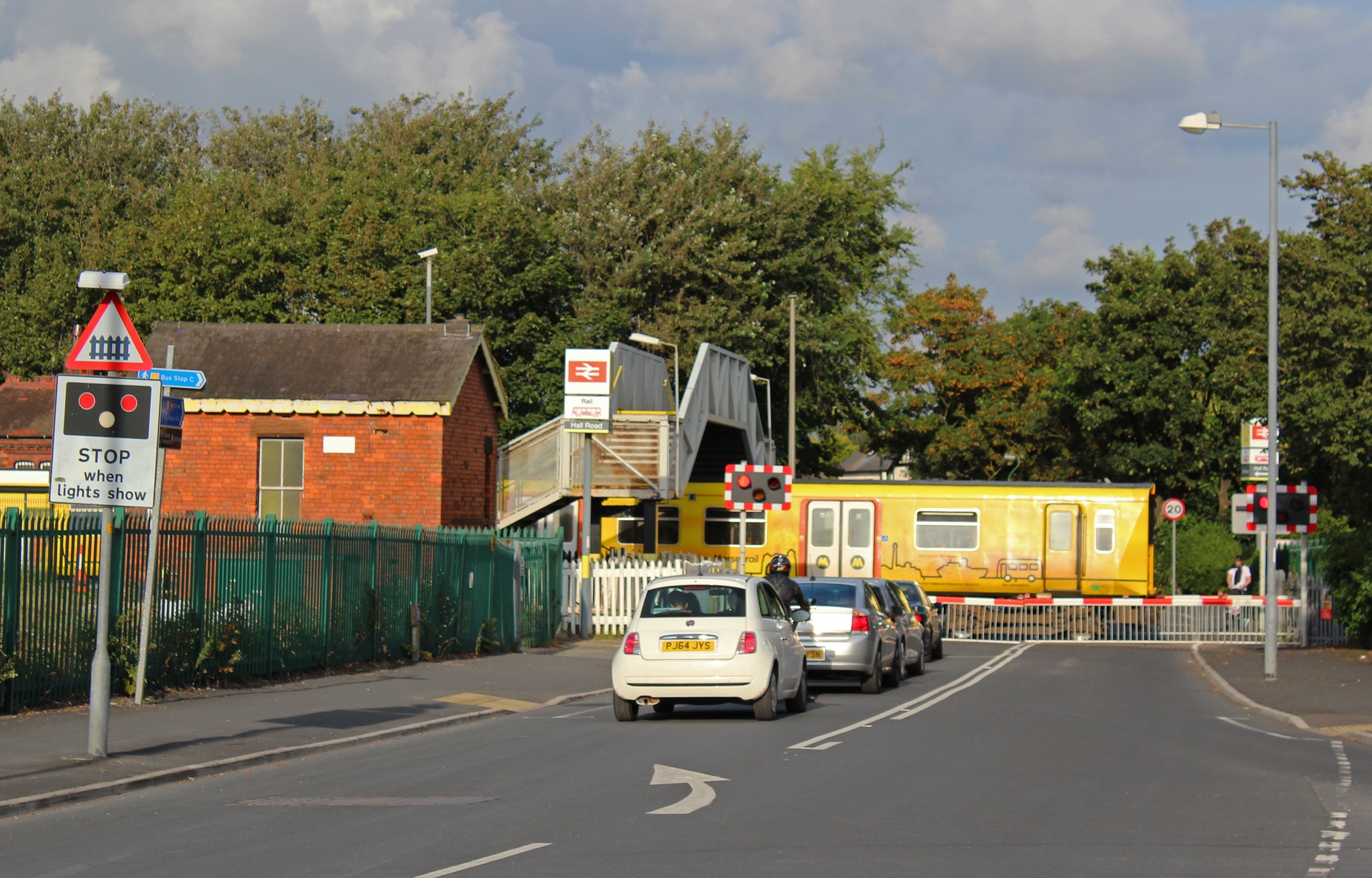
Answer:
[601, 483, 1160, 597]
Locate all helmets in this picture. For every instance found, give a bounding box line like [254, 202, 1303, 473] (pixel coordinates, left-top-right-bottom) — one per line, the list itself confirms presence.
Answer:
[770, 555, 791, 577]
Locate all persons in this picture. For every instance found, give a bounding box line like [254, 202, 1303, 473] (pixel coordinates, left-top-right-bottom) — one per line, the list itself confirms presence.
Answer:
[761, 553, 812, 629]
[668, 590, 689, 611]
[1226, 554, 1252, 593]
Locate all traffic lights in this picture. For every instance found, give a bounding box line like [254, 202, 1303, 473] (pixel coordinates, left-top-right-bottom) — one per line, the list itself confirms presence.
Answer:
[724, 462, 792, 513]
[1247, 491, 1311, 525]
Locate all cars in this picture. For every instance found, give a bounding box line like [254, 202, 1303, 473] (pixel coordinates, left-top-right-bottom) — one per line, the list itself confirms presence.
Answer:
[890, 579, 944, 662]
[845, 578, 926, 682]
[611, 575, 809, 721]
[784, 577, 899, 693]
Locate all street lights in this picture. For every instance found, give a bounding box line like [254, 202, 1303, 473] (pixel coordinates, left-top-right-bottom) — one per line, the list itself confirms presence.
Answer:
[628, 331, 680, 501]
[1178, 110, 1281, 681]
[750, 370, 773, 464]
